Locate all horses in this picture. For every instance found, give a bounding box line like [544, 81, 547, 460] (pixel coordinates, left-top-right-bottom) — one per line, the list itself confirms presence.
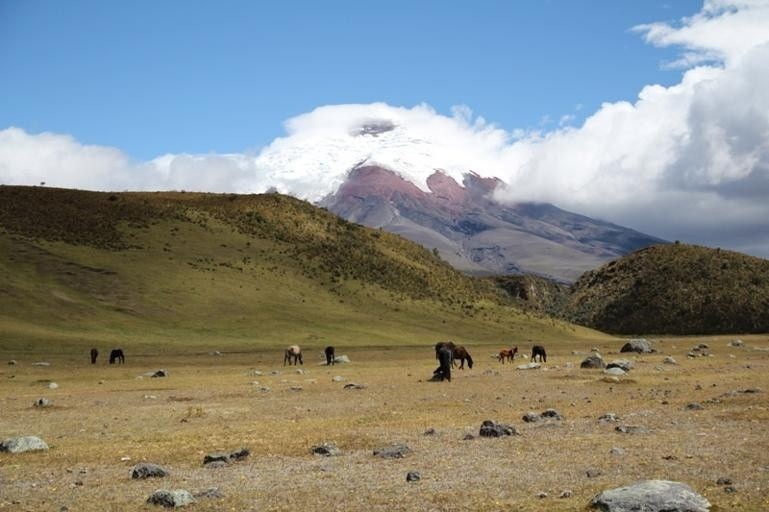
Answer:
[498, 346, 518, 364]
[283, 344, 304, 367]
[530, 345, 547, 362]
[90, 348, 98, 364]
[325, 345, 335, 365]
[435, 341, 473, 370]
[109, 348, 125, 364]
